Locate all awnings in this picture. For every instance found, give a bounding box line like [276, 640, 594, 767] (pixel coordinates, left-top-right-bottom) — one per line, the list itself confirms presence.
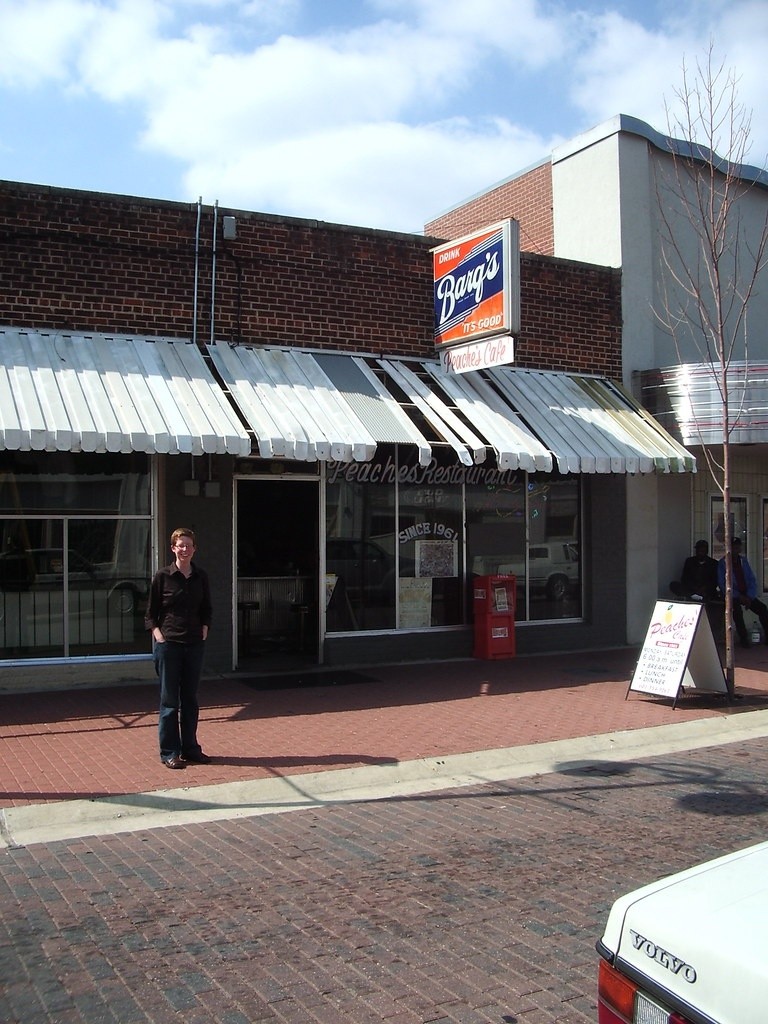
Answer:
[0, 323, 699, 478]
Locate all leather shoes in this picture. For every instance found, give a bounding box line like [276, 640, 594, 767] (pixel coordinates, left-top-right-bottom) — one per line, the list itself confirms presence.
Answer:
[161, 754, 185, 769]
[181, 744, 211, 763]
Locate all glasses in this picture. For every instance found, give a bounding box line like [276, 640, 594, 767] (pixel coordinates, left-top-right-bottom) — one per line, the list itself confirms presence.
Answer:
[174, 543, 195, 551]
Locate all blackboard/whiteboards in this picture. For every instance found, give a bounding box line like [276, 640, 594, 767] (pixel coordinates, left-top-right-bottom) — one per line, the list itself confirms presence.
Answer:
[629, 599, 729, 698]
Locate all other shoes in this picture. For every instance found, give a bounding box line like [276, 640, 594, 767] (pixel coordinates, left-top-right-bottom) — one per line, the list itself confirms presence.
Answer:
[740, 638, 753, 648]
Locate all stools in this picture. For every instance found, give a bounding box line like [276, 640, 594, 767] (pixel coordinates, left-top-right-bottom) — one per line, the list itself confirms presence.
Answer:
[290, 602, 317, 651]
[237, 601, 260, 649]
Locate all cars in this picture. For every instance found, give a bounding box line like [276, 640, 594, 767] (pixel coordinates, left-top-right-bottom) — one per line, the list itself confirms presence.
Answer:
[20, 549, 150, 619]
[591, 842, 768, 1024]
[325, 538, 438, 607]
[496, 541, 579, 601]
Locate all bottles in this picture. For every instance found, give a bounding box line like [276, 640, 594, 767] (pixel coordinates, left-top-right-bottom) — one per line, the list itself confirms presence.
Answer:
[751, 621, 760, 645]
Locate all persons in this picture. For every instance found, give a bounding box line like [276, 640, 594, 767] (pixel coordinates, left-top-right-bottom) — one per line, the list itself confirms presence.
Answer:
[145, 526, 218, 769]
[669, 540, 718, 602]
[717, 536, 768, 649]
[0, 534, 35, 658]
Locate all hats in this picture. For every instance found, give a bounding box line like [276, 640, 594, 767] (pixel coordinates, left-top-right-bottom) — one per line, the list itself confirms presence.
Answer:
[731, 537, 742, 548]
